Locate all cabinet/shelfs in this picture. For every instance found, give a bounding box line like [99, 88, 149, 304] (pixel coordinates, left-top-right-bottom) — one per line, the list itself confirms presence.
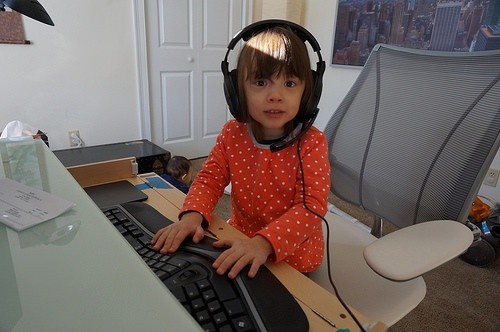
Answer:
[0, 147, 389, 332]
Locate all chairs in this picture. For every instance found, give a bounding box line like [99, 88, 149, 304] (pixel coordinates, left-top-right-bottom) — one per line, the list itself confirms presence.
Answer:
[305, 43, 500, 327]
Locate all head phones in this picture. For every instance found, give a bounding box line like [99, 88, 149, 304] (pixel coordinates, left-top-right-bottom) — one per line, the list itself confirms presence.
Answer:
[221, 19, 325, 122]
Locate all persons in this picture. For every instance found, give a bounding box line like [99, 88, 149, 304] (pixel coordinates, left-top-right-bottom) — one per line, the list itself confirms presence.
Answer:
[150, 19, 332, 278]
[160, 156, 192, 195]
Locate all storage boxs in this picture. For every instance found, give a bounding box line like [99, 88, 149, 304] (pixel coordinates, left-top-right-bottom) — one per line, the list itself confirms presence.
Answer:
[53, 139, 170, 176]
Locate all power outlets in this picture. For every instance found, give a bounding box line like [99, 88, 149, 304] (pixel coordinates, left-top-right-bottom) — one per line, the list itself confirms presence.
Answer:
[68, 131, 81, 148]
[482, 166, 499, 187]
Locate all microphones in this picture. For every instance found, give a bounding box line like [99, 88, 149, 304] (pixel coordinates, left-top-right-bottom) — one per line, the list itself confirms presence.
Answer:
[270, 108, 319, 151]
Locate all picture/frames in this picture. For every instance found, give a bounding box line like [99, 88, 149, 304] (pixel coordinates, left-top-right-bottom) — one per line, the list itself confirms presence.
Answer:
[329, 0, 500, 71]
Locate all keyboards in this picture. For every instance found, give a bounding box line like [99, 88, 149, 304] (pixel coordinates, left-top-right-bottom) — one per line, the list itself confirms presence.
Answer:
[103, 202, 309, 332]
